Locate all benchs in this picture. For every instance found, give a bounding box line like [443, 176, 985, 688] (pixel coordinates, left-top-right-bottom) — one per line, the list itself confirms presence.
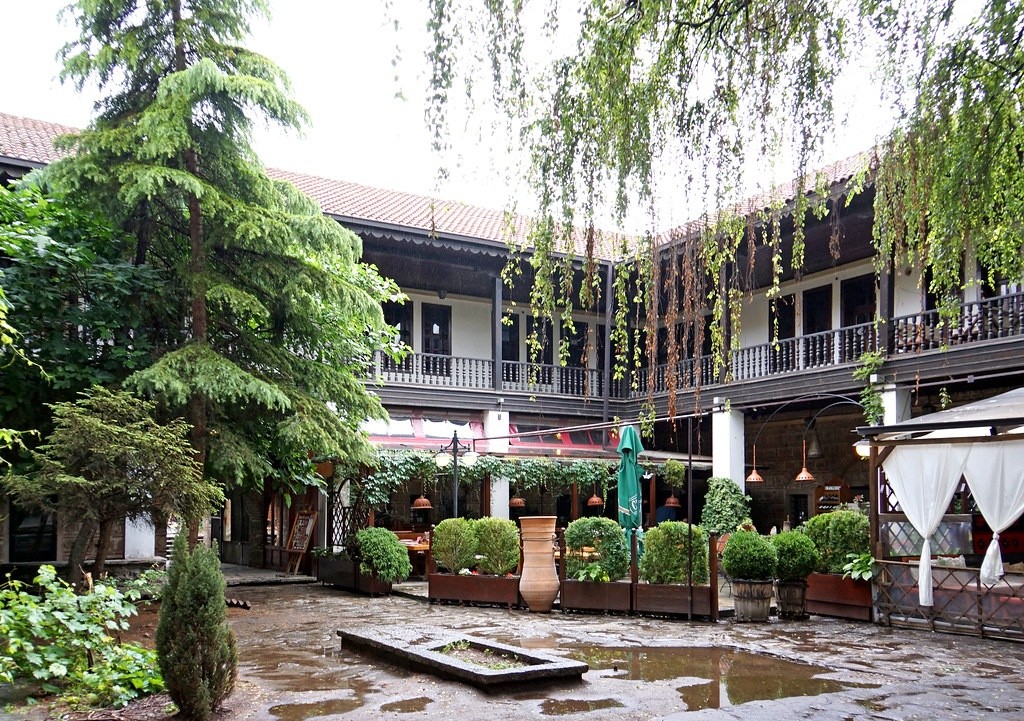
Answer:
[952, 305, 1019, 341]
[898, 321, 948, 352]
[901, 554, 971, 615]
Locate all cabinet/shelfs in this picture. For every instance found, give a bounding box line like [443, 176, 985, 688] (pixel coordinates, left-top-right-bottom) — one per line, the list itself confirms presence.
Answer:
[813, 481, 852, 516]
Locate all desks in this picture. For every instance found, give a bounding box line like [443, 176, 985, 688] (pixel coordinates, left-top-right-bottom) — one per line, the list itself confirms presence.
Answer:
[965, 581, 1023, 618]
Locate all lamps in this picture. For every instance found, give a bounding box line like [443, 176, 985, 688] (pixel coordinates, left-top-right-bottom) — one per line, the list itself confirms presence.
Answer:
[588, 483, 604, 506]
[807, 428, 824, 459]
[795, 401, 884, 482]
[921, 395, 938, 415]
[509, 496, 525, 507]
[664, 486, 682, 506]
[410, 481, 434, 509]
[804, 411, 817, 432]
[854, 436, 872, 457]
[747, 393, 880, 463]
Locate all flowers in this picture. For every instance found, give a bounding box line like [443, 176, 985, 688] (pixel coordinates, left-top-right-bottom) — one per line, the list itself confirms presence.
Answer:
[854, 495, 864, 501]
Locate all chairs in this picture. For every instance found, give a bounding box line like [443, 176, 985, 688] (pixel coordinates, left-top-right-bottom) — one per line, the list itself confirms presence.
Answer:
[998, 596, 1024, 624]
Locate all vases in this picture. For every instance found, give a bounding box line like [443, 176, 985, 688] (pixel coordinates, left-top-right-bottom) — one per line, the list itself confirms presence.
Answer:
[854, 501, 861, 508]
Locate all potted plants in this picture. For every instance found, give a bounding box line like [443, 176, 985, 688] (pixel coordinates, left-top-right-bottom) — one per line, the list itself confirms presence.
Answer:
[698, 476, 752, 554]
[428, 516, 521, 610]
[559, 516, 632, 617]
[722, 527, 781, 619]
[636, 520, 711, 623]
[364, 527, 413, 598]
[311, 545, 358, 591]
[768, 529, 819, 621]
[804, 510, 876, 622]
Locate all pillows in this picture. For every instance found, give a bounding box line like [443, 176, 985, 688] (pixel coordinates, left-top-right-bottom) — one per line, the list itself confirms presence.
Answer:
[937, 555, 973, 587]
[908, 559, 950, 593]
